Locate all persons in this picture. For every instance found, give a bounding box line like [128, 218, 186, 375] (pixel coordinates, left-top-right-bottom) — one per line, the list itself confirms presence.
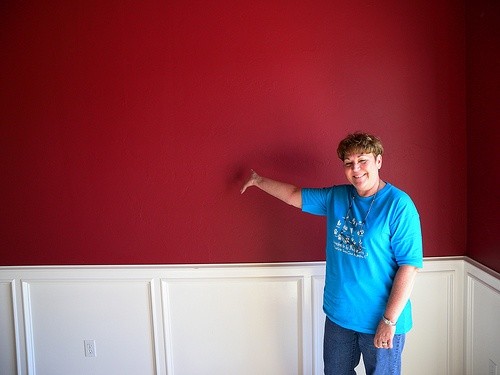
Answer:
[241, 129, 424, 375]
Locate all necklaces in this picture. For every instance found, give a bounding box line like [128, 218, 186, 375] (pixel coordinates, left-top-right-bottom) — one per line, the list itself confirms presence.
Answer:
[344, 179, 380, 226]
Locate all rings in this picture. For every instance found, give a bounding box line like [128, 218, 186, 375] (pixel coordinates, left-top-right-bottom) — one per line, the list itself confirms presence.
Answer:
[382, 341, 388, 346]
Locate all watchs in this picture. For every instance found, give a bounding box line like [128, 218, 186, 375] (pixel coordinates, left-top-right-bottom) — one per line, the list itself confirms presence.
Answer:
[382, 315, 397, 327]
